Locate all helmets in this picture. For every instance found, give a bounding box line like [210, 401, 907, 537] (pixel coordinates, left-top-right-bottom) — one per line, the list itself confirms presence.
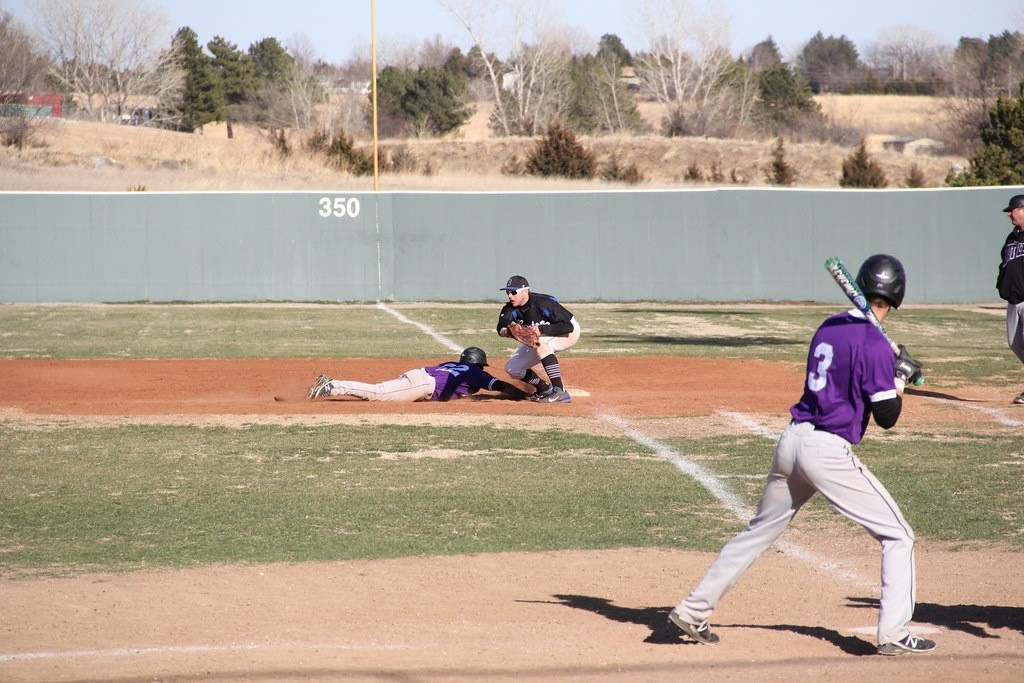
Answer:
[460, 347, 489, 366]
[855, 254, 906, 310]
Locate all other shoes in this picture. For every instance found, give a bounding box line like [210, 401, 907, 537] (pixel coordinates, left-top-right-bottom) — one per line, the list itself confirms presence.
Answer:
[1014, 392, 1024, 403]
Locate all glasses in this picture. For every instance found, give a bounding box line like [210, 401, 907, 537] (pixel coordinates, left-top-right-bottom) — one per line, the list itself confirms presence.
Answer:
[506, 290, 517, 295]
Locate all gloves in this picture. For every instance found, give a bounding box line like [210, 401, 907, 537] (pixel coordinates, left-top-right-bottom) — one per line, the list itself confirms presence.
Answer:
[895, 344, 924, 385]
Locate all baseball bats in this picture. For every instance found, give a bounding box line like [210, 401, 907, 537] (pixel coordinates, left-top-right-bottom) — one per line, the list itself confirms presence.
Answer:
[824, 255, 925, 386]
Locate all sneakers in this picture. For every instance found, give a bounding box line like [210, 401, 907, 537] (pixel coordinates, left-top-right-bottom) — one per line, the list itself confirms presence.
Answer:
[308, 374, 334, 400]
[877, 634, 937, 655]
[526, 391, 554, 401]
[669, 610, 721, 646]
[538, 390, 571, 403]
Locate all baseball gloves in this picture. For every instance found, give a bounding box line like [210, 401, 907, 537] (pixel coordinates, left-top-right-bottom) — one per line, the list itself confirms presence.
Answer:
[506, 320, 541, 350]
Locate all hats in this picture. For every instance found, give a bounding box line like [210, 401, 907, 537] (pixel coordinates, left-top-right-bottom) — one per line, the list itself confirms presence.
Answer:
[1002, 194, 1024, 212]
[501, 275, 529, 291]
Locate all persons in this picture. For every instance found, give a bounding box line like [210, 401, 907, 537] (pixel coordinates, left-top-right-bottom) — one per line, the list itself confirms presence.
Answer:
[996, 195, 1024, 403]
[668, 254, 936, 654]
[497, 276, 580, 403]
[309, 347, 536, 402]
